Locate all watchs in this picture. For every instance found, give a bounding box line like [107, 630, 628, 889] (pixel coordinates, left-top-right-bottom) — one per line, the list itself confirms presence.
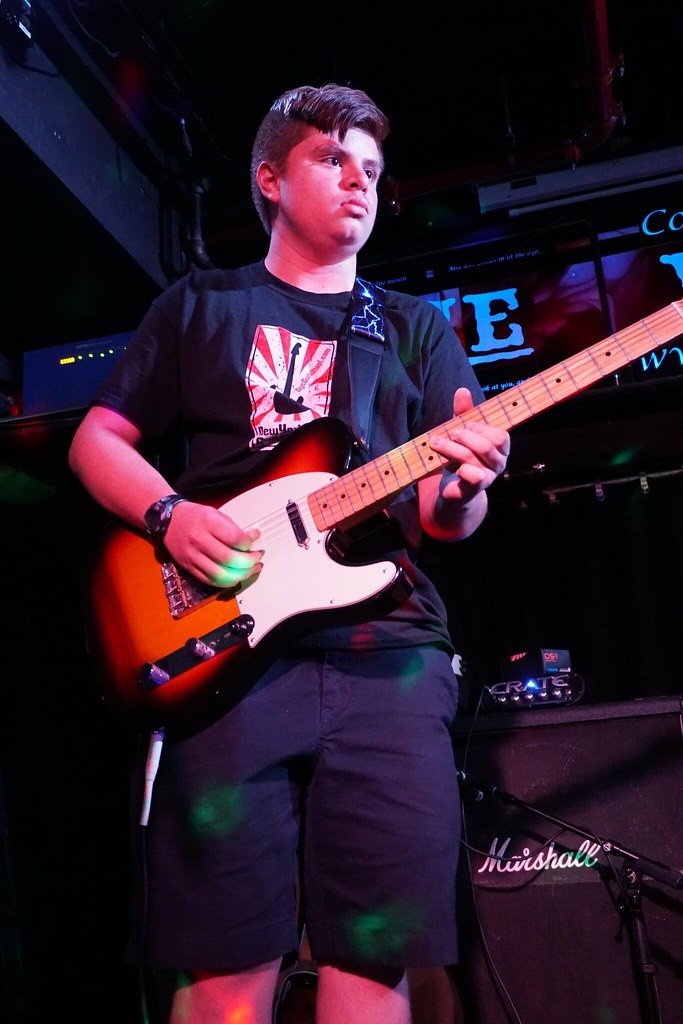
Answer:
[143, 494, 187, 550]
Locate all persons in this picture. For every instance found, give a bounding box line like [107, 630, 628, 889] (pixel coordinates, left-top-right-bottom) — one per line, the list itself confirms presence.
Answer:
[67, 86, 509, 1024]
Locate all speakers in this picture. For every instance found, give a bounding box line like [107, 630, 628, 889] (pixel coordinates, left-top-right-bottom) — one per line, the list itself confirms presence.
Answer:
[449, 694, 683, 1024]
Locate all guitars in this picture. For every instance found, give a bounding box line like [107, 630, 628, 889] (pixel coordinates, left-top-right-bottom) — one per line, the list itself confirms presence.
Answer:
[84, 297, 683, 744]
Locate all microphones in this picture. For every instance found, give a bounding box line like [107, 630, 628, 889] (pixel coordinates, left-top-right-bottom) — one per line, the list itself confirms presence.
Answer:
[457, 781, 483, 802]
